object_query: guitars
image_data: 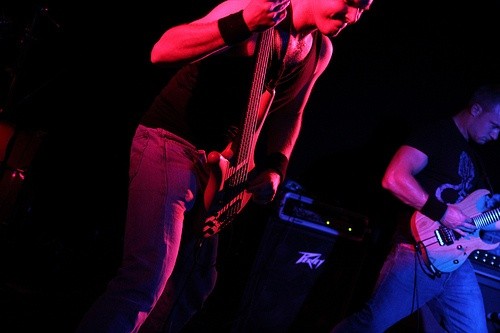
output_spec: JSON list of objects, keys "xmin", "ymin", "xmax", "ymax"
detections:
[
  {"xmin": 200, "ymin": 26, "xmax": 275, "ymax": 240},
  {"xmin": 410, "ymin": 189, "xmax": 500, "ymax": 274}
]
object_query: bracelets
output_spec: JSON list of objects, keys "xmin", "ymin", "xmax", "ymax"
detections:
[
  {"xmin": 217, "ymin": 10, "xmax": 253, "ymax": 47},
  {"xmin": 419, "ymin": 194, "xmax": 449, "ymax": 222},
  {"xmin": 262, "ymin": 151, "xmax": 289, "ymax": 182}
]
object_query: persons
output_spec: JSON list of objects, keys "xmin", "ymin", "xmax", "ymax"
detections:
[
  {"xmin": 330, "ymin": 79, "xmax": 500, "ymax": 333},
  {"xmin": 100, "ymin": 0, "xmax": 377, "ymax": 333}
]
